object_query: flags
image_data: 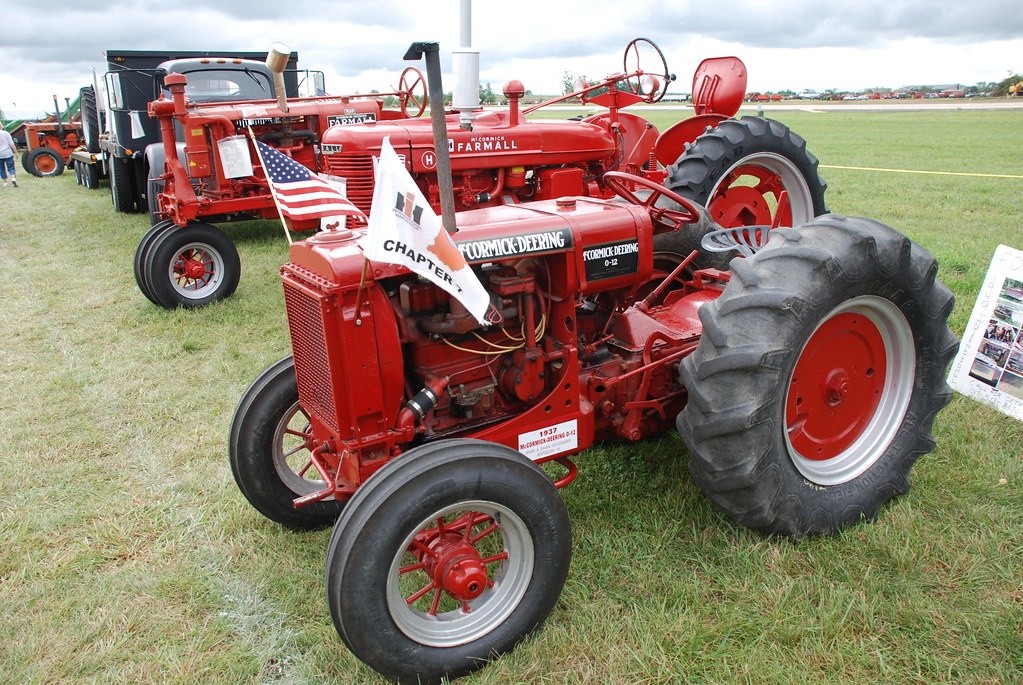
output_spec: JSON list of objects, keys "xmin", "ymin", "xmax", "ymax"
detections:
[
  {"xmin": 253, "ymin": 140, "xmax": 367, "ymax": 220},
  {"xmin": 363, "ymin": 136, "xmax": 492, "ymax": 327}
]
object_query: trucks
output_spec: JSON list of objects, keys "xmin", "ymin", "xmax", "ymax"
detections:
[{"xmin": 67, "ymin": 48, "xmax": 325, "ymax": 214}]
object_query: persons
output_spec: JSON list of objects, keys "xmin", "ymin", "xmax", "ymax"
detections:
[{"xmin": 0, "ymin": 120, "xmax": 20, "ymax": 189}]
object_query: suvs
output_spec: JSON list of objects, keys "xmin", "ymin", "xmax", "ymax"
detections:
[{"xmin": 132, "ymin": 51, "xmax": 429, "ymax": 311}]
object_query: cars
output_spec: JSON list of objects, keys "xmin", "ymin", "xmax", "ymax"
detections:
[{"xmin": 744, "ymin": 80, "xmax": 993, "ymax": 103}]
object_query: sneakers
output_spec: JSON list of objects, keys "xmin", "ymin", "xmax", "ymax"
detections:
[
  {"xmin": 3, "ymin": 183, "xmax": 9, "ymax": 187},
  {"xmin": 12, "ymin": 178, "xmax": 18, "ymax": 187}
]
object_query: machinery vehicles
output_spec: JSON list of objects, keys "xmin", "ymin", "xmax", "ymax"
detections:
[{"xmin": 226, "ymin": 36, "xmax": 962, "ymax": 684}]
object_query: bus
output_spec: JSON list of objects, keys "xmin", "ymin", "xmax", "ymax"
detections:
[{"xmin": 20, "ymin": 94, "xmax": 88, "ymax": 179}]
